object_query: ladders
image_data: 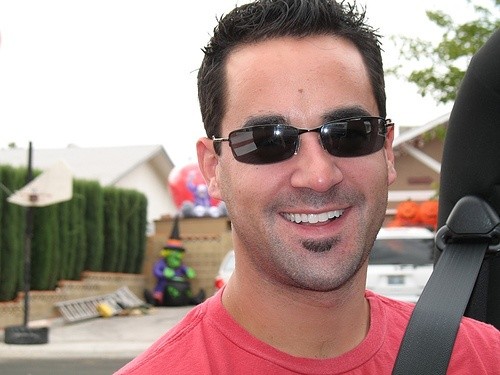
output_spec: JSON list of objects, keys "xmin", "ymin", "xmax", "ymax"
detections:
[{"xmin": 55, "ymin": 288, "xmax": 148, "ymax": 323}]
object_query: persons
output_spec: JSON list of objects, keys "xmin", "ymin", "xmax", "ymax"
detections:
[{"xmin": 110, "ymin": 0, "xmax": 500, "ymax": 375}]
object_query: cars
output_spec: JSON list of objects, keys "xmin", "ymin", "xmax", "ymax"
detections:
[{"xmin": 212, "ymin": 224, "xmax": 444, "ymax": 305}]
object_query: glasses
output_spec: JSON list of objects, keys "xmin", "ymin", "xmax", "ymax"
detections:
[{"xmin": 212, "ymin": 116, "xmax": 392, "ymax": 164}]
